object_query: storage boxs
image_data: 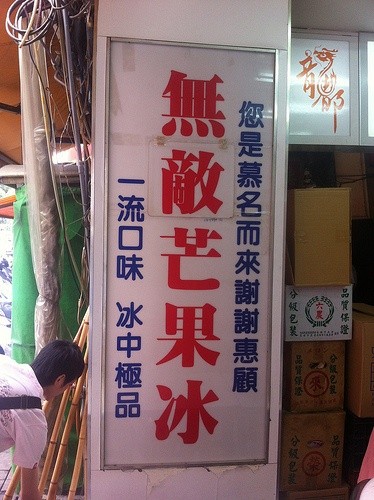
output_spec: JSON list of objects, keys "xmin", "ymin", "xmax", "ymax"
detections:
[{"xmin": 277, "ymin": 152, "xmax": 374, "ymax": 500}]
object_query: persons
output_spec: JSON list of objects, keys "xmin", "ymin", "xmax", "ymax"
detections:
[{"xmin": 0, "ymin": 339, "xmax": 85, "ymax": 500}]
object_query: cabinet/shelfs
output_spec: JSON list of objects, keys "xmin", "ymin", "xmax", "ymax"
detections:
[{"xmin": 288, "ymin": 25, "xmax": 374, "ymax": 147}]
